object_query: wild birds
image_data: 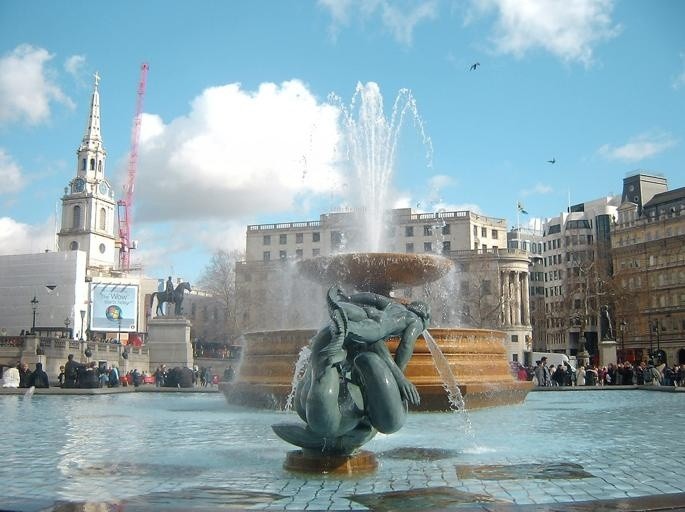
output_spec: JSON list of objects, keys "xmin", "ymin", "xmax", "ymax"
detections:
[
  {"xmin": 548, "ymin": 156, "xmax": 556, "ymax": 164},
  {"xmin": 469, "ymin": 62, "xmax": 480, "ymax": 72}
]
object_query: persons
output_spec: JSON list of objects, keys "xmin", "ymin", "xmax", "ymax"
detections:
[
  {"xmin": 0, "ymin": 327, "xmax": 241, "ymax": 388},
  {"xmin": 337, "ymin": 287, "xmax": 433, "ymax": 405},
  {"xmin": 166, "ymin": 276, "xmax": 175, "ymax": 304},
  {"xmin": 510, "ymin": 356, "xmax": 685, "ymax": 388},
  {"xmin": 601, "ymin": 304, "xmax": 616, "ymax": 341}
]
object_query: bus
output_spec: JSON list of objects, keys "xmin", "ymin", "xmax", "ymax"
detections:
[{"xmin": 591, "ymin": 346, "xmax": 642, "ymax": 365}]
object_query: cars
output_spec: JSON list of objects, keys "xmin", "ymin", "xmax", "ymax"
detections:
[{"xmin": 509, "ymin": 361, "xmax": 528, "ymax": 371}]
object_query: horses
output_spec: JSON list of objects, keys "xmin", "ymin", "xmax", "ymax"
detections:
[{"xmin": 150, "ymin": 281, "xmax": 192, "ymax": 316}]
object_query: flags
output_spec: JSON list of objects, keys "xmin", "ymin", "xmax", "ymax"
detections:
[{"xmin": 518, "ymin": 203, "xmax": 529, "ymax": 214}]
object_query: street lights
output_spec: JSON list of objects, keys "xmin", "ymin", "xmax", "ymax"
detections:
[
  {"xmin": 651, "ymin": 326, "xmax": 663, "ymax": 365},
  {"xmin": 618, "ymin": 317, "xmax": 626, "ymax": 363},
  {"xmin": 62, "ymin": 314, "xmax": 71, "ymax": 338},
  {"xmin": 78, "ymin": 307, "xmax": 87, "ymax": 340},
  {"xmin": 191, "ymin": 335, "xmax": 198, "ymax": 366},
  {"xmin": 115, "ymin": 310, "xmax": 123, "ymax": 342},
  {"xmin": 29, "ymin": 294, "xmax": 40, "ymax": 337},
  {"xmin": 647, "ymin": 318, "xmax": 655, "ymax": 364}
]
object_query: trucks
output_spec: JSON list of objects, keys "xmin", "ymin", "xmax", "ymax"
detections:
[{"xmin": 528, "ymin": 350, "xmax": 576, "ymax": 371}]
